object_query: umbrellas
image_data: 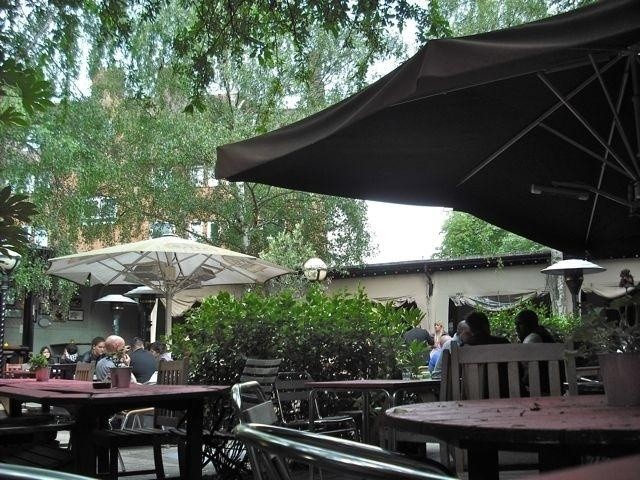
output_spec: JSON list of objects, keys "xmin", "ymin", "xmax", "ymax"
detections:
[
  {"xmin": 42, "ymin": 229, "xmax": 299, "ymax": 344},
  {"xmin": 214, "ymin": 0, "xmax": 640, "ymax": 262}
]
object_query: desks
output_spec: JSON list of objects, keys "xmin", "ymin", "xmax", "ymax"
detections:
[{"xmin": 383, "ymin": 395, "xmax": 640, "ymax": 480}]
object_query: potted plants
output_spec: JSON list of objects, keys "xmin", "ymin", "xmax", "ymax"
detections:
[{"xmin": 567, "ymin": 306, "xmax": 640, "ymax": 405}]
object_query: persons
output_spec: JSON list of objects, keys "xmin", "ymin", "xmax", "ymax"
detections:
[
  {"xmin": 38, "ymin": 335, "xmax": 176, "ymax": 389},
  {"xmin": 431, "ymin": 319, "xmax": 472, "ymax": 378},
  {"xmin": 460, "ymin": 313, "xmax": 524, "ymax": 400},
  {"xmin": 403, "ymin": 324, "xmax": 434, "ymax": 351},
  {"xmin": 429, "ymin": 336, "xmax": 450, "ymax": 373},
  {"xmin": 514, "ymin": 309, "xmax": 566, "ymax": 397},
  {"xmin": 433, "ymin": 321, "xmax": 451, "ymax": 352}
]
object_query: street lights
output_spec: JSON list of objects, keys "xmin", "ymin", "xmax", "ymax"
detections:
[
  {"xmin": 0, "ymin": 245, "xmax": 22, "ymax": 355},
  {"xmin": 541, "ymin": 257, "xmax": 608, "ymax": 324},
  {"xmin": 21, "ymin": 225, "xmax": 48, "ymax": 349}
]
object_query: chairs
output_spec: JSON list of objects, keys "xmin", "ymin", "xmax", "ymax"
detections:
[
  {"xmin": 380, "ymin": 349, "xmax": 450, "ymax": 470},
  {"xmin": 451, "ymin": 340, "xmax": 577, "ymax": 480}
]
